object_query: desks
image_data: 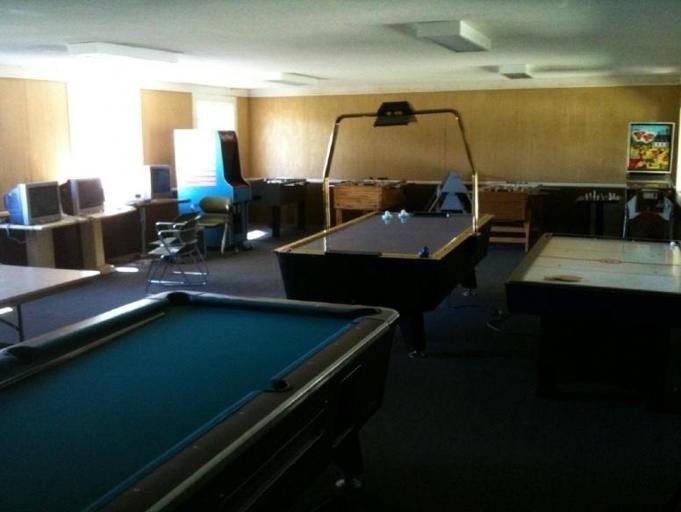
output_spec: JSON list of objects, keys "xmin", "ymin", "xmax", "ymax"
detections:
[
  {"xmin": 128, "ymin": 198, "xmax": 189, "ymax": 243},
  {"xmin": 80, "ymin": 206, "xmax": 136, "ymax": 274},
  {"xmin": 0, "ymin": 215, "xmax": 87, "ymax": 269},
  {"xmin": 0, "ymin": 262, "xmax": 101, "ymax": 349}
]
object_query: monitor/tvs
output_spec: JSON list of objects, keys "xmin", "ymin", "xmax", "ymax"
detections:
[
  {"xmin": 2, "ymin": 180, "xmax": 63, "ymax": 226},
  {"xmin": 141, "ymin": 165, "xmax": 172, "ymax": 200},
  {"xmin": 59, "ymin": 177, "xmax": 105, "ymax": 216}
]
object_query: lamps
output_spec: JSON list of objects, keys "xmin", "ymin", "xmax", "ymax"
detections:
[{"xmin": 68, "ymin": 42, "xmax": 180, "ymax": 69}]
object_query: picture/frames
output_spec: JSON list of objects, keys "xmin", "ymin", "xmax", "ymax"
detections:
[{"xmin": 626, "ymin": 122, "xmax": 676, "ymax": 174}]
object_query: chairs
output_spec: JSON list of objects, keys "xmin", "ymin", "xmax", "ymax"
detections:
[
  {"xmin": 190, "ymin": 196, "xmax": 239, "ymax": 255},
  {"xmin": 147, "ymin": 213, "xmax": 210, "ymax": 274},
  {"xmin": 145, "ymin": 215, "xmax": 210, "ymax": 290}
]
